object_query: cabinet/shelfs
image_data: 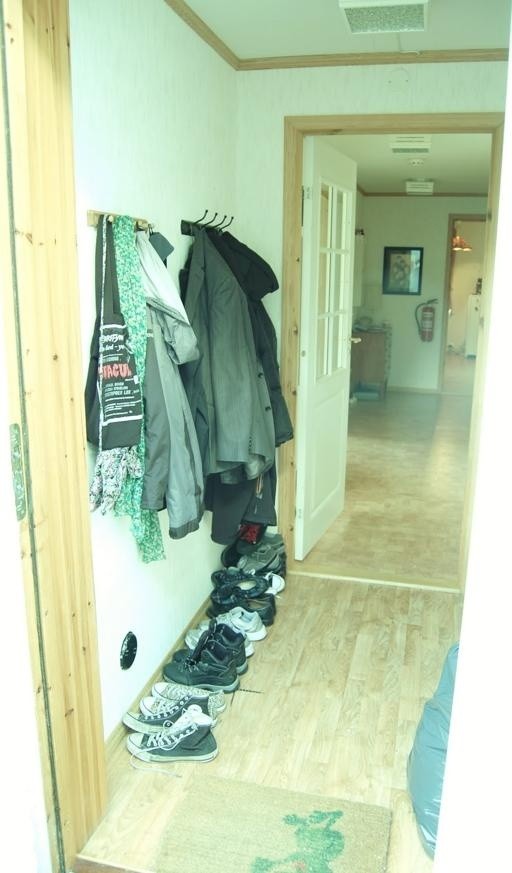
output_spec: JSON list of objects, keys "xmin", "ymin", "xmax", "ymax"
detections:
[{"xmin": 350, "ymin": 331, "xmax": 391, "ymax": 399}]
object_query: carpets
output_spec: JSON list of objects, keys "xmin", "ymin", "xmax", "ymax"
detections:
[{"xmin": 150, "ymin": 774, "xmax": 392, "ymax": 873}]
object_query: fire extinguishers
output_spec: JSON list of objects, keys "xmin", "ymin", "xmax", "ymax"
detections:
[{"xmin": 415, "ymin": 299, "xmax": 438, "ymax": 342}]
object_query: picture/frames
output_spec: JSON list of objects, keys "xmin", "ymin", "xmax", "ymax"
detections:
[{"xmin": 382, "ymin": 247, "xmax": 423, "ymax": 295}]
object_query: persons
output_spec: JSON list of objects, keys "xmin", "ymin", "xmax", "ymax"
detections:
[{"xmin": 392, "ymin": 255, "xmax": 410, "ymax": 289}]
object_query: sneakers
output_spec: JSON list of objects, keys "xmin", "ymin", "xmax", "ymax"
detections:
[{"xmin": 122, "ymin": 523, "xmax": 287, "ymax": 763}]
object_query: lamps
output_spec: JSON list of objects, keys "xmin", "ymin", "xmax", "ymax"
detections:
[{"xmin": 452, "ymin": 235, "xmax": 472, "ymax": 252}]
